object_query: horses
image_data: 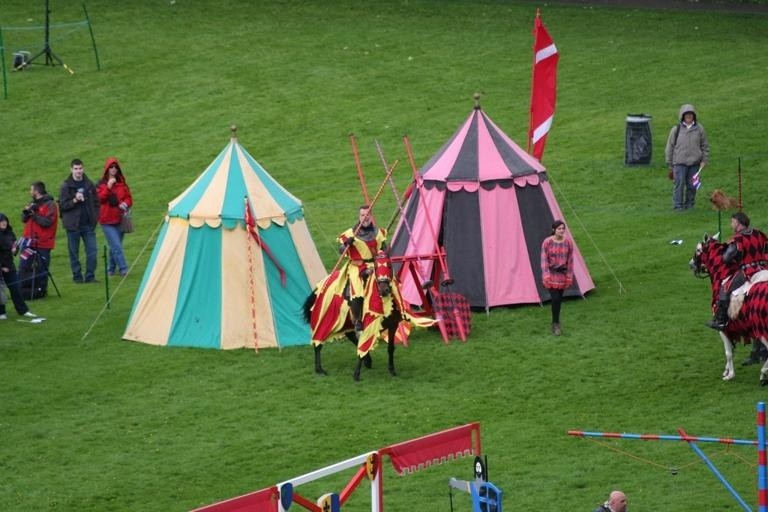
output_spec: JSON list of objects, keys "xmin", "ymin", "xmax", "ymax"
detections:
[
  {"xmin": 689, "ymin": 231, "xmax": 768, "ymax": 386},
  {"xmin": 293, "ymin": 243, "xmax": 402, "ymax": 381}
]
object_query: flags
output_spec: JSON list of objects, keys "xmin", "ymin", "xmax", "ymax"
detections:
[
  {"xmin": 530, "ymin": 18, "xmax": 559, "ymax": 162},
  {"xmin": 693, "ymin": 174, "xmax": 702, "ymax": 190},
  {"xmin": 20, "ymin": 248, "xmax": 34, "ymax": 261},
  {"xmin": 18, "ymin": 237, "xmax": 32, "ymax": 247}
]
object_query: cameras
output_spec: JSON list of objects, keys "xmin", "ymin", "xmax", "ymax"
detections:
[{"xmin": 22, "ymin": 203, "xmax": 36, "ymax": 214}]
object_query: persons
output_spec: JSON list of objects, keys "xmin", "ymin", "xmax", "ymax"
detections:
[
  {"xmin": 705, "ymin": 213, "xmax": 768, "ymax": 331},
  {"xmin": 595, "ymin": 490, "xmax": 627, "ymax": 512},
  {"xmin": 94, "ymin": 157, "xmax": 133, "ymax": 277},
  {"xmin": 339, "ymin": 205, "xmax": 389, "ymax": 330},
  {"xmin": 58, "ymin": 159, "xmax": 100, "ymax": 285},
  {"xmin": 664, "ymin": 105, "xmax": 710, "ymax": 212},
  {"xmin": 17, "ymin": 181, "xmax": 58, "ymax": 297},
  {"xmin": 0, "ymin": 214, "xmax": 36, "ymax": 320},
  {"xmin": 540, "ymin": 220, "xmax": 574, "ymax": 336}
]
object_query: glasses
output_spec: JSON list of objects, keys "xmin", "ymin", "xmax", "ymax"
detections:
[{"xmin": 109, "ymin": 164, "xmax": 119, "ymax": 169}]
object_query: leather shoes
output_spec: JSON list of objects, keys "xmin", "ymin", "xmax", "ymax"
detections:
[{"xmin": 741, "ymin": 360, "xmax": 759, "ymax": 366}]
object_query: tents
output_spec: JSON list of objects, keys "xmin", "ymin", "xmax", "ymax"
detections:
[
  {"xmin": 387, "ymin": 94, "xmax": 597, "ymax": 308},
  {"xmin": 120, "ymin": 125, "xmax": 330, "ymax": 351}
]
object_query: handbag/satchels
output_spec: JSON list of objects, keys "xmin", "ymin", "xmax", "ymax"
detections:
[{"xmin": 119, "ymin": 208, "xmax": 134, "ymax": 234}]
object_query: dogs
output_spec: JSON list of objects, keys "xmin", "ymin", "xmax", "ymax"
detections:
[{"xmin": 709, "ymin": 189, "xmax": 743, "ymax": 210}]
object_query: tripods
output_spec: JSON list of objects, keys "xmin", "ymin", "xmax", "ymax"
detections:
[
  {"xmin": 30, "ymin": 214, "xmax": 61, "ymax": 300},
  {"xmin": 11, "ymin": 0, "xmax": 75, "ymax": 74}
]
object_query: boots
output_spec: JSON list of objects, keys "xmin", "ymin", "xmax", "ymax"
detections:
[{"xmin": 711, "ymin": 300, "xmax": 727, "ymax": 327}]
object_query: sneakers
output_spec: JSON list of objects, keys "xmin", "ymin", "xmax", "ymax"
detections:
[
  {"xmin": 0, "ymin": 314, "xmax": 6, "ymax": 320},
  {"xmin": 553, "ymin": 323, "xmax": 560, "ymax": 336},
  {"xmin": 23, "ymin": 312, "xmax": 36, "ymax": 317},
  {"xmin": 73, "ymin": 275, "xmax": 82, "ymax": 281},
  {"xmin": 120, "ymin": 271, "xmax": 126, "ymax": 276},
  {"xmin": 108, "ymin": 271, "xmax": 113, "ymax": 276},
  {"xmin": 85, "ymin": 275, "xmax": 93, "ymax": 282}
]
